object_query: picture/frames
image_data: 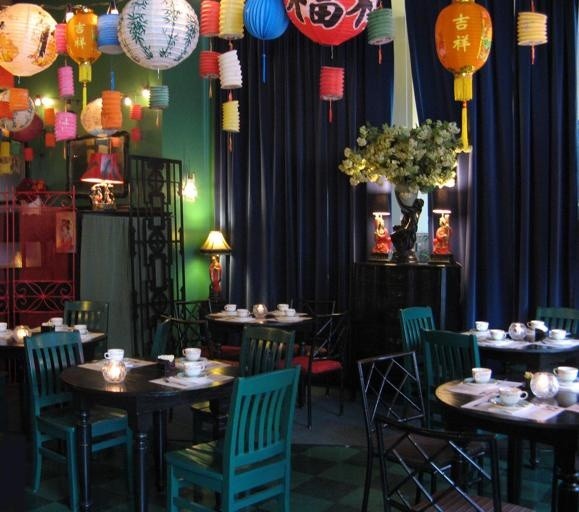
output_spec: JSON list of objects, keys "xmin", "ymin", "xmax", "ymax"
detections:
[{"xmin": 65, "ymin": 131, "xmax": 129, "ymax": 200}]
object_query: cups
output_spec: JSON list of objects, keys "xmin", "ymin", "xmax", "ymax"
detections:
[
  {"xmin": 552, "ymin": 366, "xmax": 579, "ymax": 383},
  {"xmin": 224, "ymin": 304, "xmax": 296, "ymax": 317},
  {"xmin": 0, "ymin": 317, "xmax": 87, "ymax": 337},
  {"xmin": 183, "ymin": 347, "xmax": 201, "ymax": 361},
  {"xmin": 472, "ymin": 368, "xmax": 491, "ymax": 383},
  {"xmin": 475, "ymin": 320, "xmax": 567, "ymax": 340},
  {"xmin": 104, "ymin": 348, "xmax": 125, "ymax": 362},
  {"xmin": 497, "ymin": 385, "xmax": 528, "ymax": 405},
  {"xmin": 184, "ymin": 361, "xmax": 205, "ymax": 376}
]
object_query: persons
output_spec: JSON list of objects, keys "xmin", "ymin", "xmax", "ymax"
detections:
[
  {"xmin": 208, "ymin": 256, "xmax": 223, "ymax": 298},
  {"xmin": 434, "ymin": 215, "xmax": 452, "ymax": 253},
  {"xmin": 374, "ymin": 216, "xmax": 391, "ymax": 254}
]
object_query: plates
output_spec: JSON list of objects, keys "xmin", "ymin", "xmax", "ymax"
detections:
[
  {"xmin": 177, "ymin": 372, "xmax": 206, "ymax": 380},
  {"xmin": 489, "ymin": 395, "xmax": 531, "ymax": 410},
  {"xmin": 464, "ymin": 377, "xmax": 497, "ymax": 388}
]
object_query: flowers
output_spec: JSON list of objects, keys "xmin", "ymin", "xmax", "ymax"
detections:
[{"xmin": 339, "ymin": 118, "xmax": 464, "ymax": 194}]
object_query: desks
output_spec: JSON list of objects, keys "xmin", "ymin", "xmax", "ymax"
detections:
[{"xmin": 347, "ymin": 261, "xmax": 465, "ymax": 404}]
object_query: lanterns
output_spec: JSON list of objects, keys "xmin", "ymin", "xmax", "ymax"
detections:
[{"xmin": 0, "ymin": 1, "xmax": 557, "ymax": 154}]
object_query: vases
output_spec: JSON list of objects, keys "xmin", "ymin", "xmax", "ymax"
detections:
[{"xmin": 394, "ymin": 176, "xmax": 417, "ymax": 208}]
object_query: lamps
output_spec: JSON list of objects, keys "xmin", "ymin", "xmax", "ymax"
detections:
[
  {"xmin": 34, "ymin": 94, "xmax": 41, "ymax": 105},
  {"xmin": 124, "ymin": 96, "xmax": 132, "ymax": 106},
  {"xmin": 143, "ymin": 87, "xmax": 151, "ymax": 98},
  {"xmin": 199, "ymin": 230, "xmax": 232, "ymax": 302},
  {"xmin": 64, "ymin": 99, "xmax": 72, "ymax": 112},
  {"xmin": 80, "ymin": 152, "xmax": 127, "ymax": 211},
  {"xmin": 182, "ymin": 173, "xmax": 199, "ymax": 203},
  {"xmin": 111, "ymin": 1, "xmax": 119, "ymax": 14},
  {"xmin": 427, "ymin": 188, "xmax": 454, "ymax": 264},
  {"xmin": 65, "ymin": 3, "xmax": 74, "ymax": 24},
  {"xmin": 368, "ymin": 194, "xmax": 392, "ymax": 262}
]
